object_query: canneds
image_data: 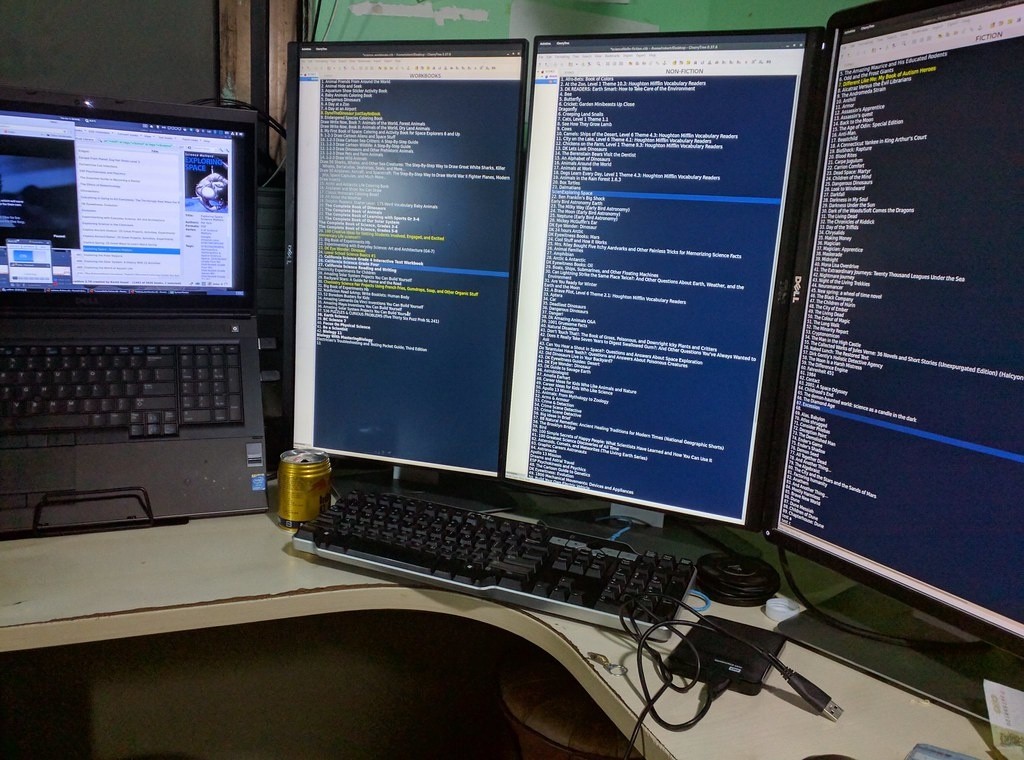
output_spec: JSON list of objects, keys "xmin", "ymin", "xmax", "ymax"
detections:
[{"xmin": 276, "ymin": 449, "xmax": 332, "ymax": 533}]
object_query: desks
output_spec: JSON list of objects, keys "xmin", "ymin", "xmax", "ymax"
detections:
[{"xmin": 0, "ymin": 466, "xmax": 1005, "ymax": 760}]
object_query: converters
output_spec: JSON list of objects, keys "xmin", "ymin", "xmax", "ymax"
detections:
[{"xmin": 668, "ymin": 615, "xmax": 788, "ymax": 697}]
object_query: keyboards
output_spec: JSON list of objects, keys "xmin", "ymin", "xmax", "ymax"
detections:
[{"xmin": 291, "ymin": 488, "xmax": 698, "ymax": 642}]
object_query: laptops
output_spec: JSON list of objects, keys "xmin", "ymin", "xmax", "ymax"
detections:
[{"xmin": 0, "ymin": 83, "xmax": 271, "ymax": 541}]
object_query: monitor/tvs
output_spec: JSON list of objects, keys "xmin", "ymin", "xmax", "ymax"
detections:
[
  {"xmin": 499, "ymin": 25, "xmax": 827, "ymax": 534},
  {"xmin": 282, "ymin": 38, "xmax": 530, "ymax": 481},
  {"xmin": 759, "ymin": 0, "xmax": 1024, "ymax": 662}
]
